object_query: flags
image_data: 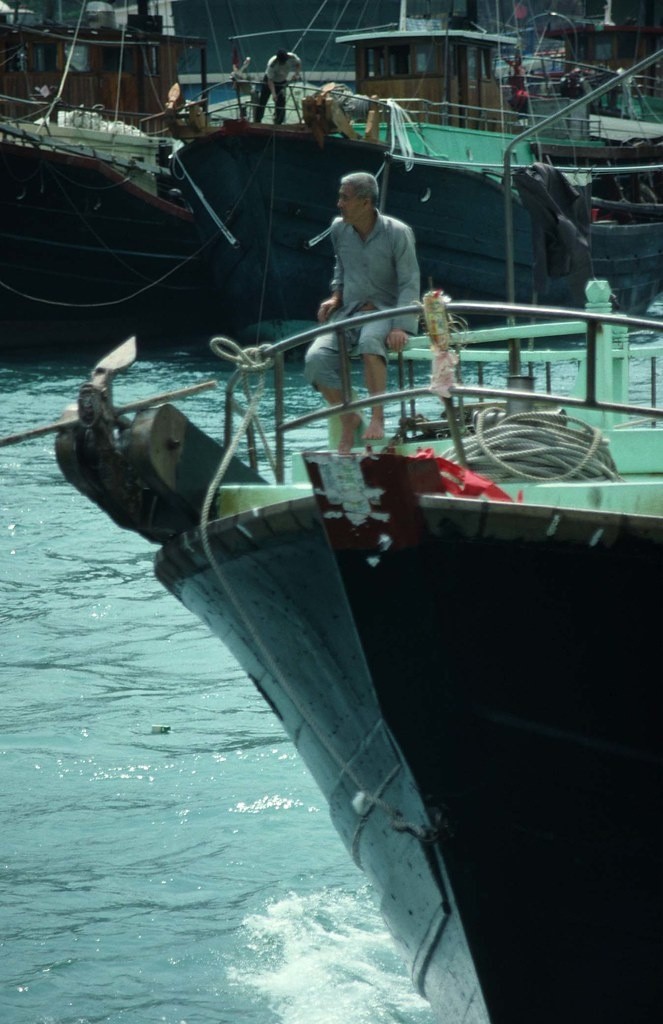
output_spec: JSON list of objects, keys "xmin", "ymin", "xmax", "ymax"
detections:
[{"xmin": 514, "ymin": 3, "xmax": 526, "ymax": 18}]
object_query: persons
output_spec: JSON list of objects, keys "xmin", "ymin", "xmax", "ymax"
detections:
[
  {"xmin": 254, "ymin": 48, "xmax": 301, "ymax": 125},
  {"xmin": 503, "ymin": 56, "xmax": 523, "ymax": 74},
  {"xmin": 305, "ymin": 172, "xmax": 420, "ymax": 454}
]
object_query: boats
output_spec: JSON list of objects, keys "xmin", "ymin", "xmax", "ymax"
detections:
[
  {"xmin": 55, "ymin": 301, "xmax": 663, "ymax": 1024},
  {"xmin": 0, "ymin": 0, "xmax": 355, "ymax": 345},
  {"xmin": 169, "ymin": 0, "xmax": 663, "ymax": 357}
]
